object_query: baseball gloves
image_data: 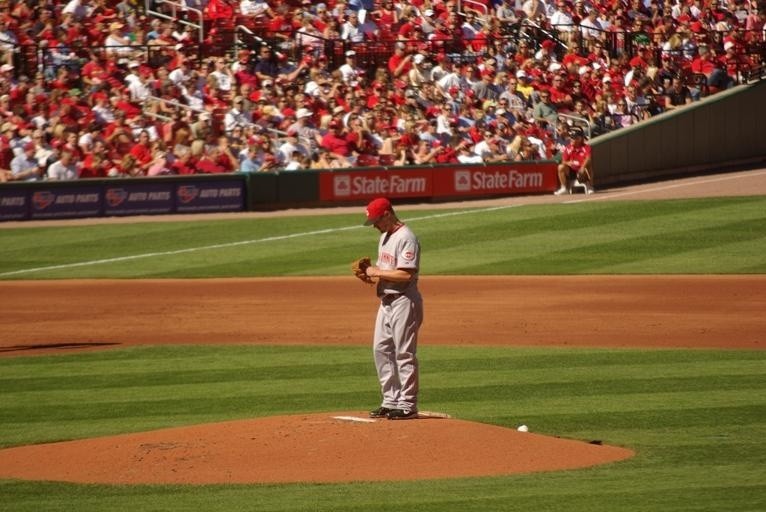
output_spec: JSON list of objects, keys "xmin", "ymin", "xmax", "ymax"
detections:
[
  {"xmin": 351, "ymin": 256, "xmax": 376, "ymax": 284},
  {"xmin": 577, "ymin": 168, "xmax": 590, "ymax": 185}
]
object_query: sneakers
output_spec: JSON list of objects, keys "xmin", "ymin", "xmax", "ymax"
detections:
[
  {"xmin": 369, "ymin": 406, "xmax": 396, "ymax": 418},
  {"xmin": 588, "ymin": 188, "xmax": 595, "ymax": 194},
  {"xmin": 386, "ymin": 410, "xmax": 419, "ymax": 420},
  {"xmin": 554, "ymin": 189, "xmax": 568, "ymax": 196}
]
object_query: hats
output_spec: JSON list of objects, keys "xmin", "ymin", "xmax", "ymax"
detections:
[
  {"xmin": 362, "ymin": 197, "xmax": 392, "ymax": 226},
  {"xmin": 1, "ymin": 0, "xmax": 736, "ymax": 157}
]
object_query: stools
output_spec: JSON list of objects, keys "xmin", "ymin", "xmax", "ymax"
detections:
[{"xmin": 568, "ymin": 174, "xmax": 593, "ymax": 195}]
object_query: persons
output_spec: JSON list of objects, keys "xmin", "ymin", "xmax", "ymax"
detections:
[
  {"xmin": 0, "ymin": 1, "xmax": 765, "ymax": 182},
  {"xmin": 354, "ymin": 198, "xmax": 424, "ymax": 420},
  {"xmin": 552, "ymin": 125, "xmax": 594, "ymax": 195}
]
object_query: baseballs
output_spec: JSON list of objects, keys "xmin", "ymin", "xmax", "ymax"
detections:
[{"xmin": 517, "ymin": 425, "xmax": 528, "ymax": 432}]
